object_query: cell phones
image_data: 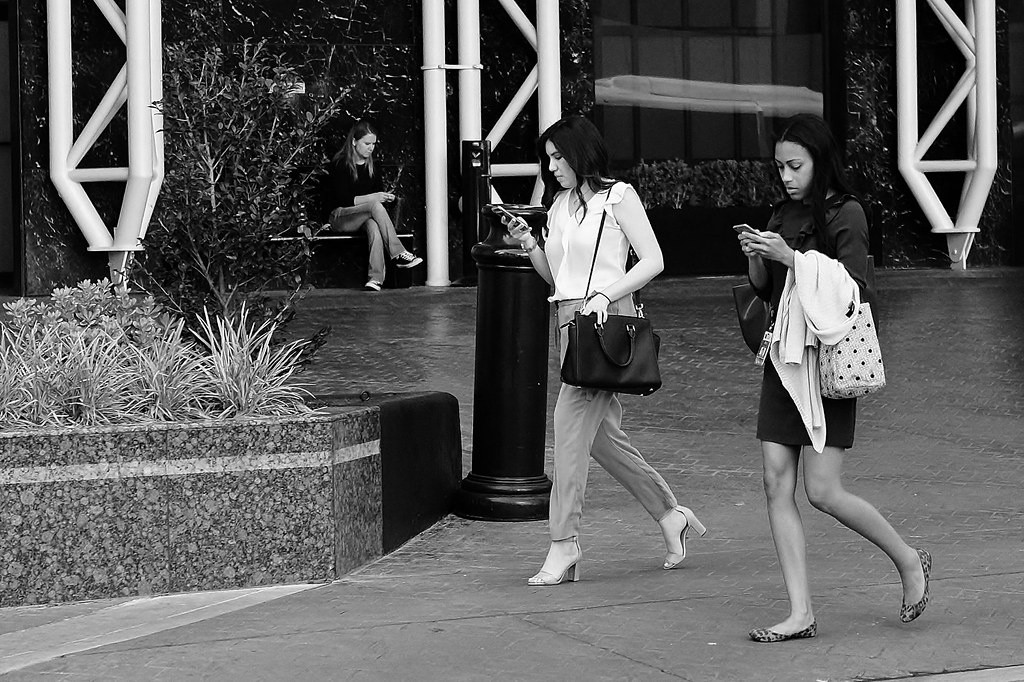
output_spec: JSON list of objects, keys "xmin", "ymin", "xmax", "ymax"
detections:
[
  {"xmin": 733, "ymin": 224, "xmax": 761, "ymax": 237},
  {"xmin": 491, "ymin": 206, "xmax": 529, "ymax": 231}
]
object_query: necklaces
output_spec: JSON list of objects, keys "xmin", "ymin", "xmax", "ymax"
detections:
[{"xmin": 571, "ymin": 189, "xmax": 592, "ymax": 204}]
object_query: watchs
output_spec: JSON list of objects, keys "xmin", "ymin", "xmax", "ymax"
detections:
[{"xmin": 520, "ymin": 238, "xmax": 537, "ymax": 252}]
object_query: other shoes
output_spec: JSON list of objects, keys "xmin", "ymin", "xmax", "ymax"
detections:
[
  {"xmin": 748, "ymin": 618, "xmax": 817, "ymax": 642},
  {"xmin": 899, "ymin": 548, "xmax": 931, "ymax": 623}
]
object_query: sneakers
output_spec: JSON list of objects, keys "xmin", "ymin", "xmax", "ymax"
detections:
[
  {"xmin": 365, "ymin": 279, "xmax": 382, "ymax": 291},
  {"xmin": 396, "ymin": 250, "xmax": 424, "ymax": 269}
]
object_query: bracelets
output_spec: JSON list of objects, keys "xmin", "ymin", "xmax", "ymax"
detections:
[{"xmin": 598, "ymin": 292, "xmax": 612, "ymax": 304}]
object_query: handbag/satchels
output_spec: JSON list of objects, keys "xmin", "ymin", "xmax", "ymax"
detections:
[
  {"xmin": 820, "ymin": 299, "xmax": 887, "ymax": 400},
  {"xmin": 732, "ymin": 283, "xmax": 770, "ymax": 355},
  {"xmin": 560, "ymin": 309, "xmax": 663, "ymax": 396}
]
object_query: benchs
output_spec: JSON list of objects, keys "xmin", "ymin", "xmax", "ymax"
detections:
[{"xmin": 191, "ymin": 232, "xmax": 416, "ymax": 289}]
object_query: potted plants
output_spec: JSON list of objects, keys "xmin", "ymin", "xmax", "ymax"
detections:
[{"xmin": 629, "ymin": 158, "xmax": 884, "ymax": 279}]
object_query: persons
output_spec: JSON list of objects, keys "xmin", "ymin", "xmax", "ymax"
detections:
[
  {"xmin": 500, "ymin": 118, "xmax": 707, "ymax": 586},
  {"xmin": 737, "ymin": 112, "xmax": 932, "ymax": 643},
  {"xmin": 322, "ymin": 122, "xmax": 424, "ymax": 292}
]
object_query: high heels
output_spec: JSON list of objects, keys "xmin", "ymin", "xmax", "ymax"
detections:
[
  {"xmin": 662, "ymin": 505, "xmax": 708, "ymax": 570},
  {"xmin": 527, "ymin": 537, "xmax": 583, "ymax": 585}
]
